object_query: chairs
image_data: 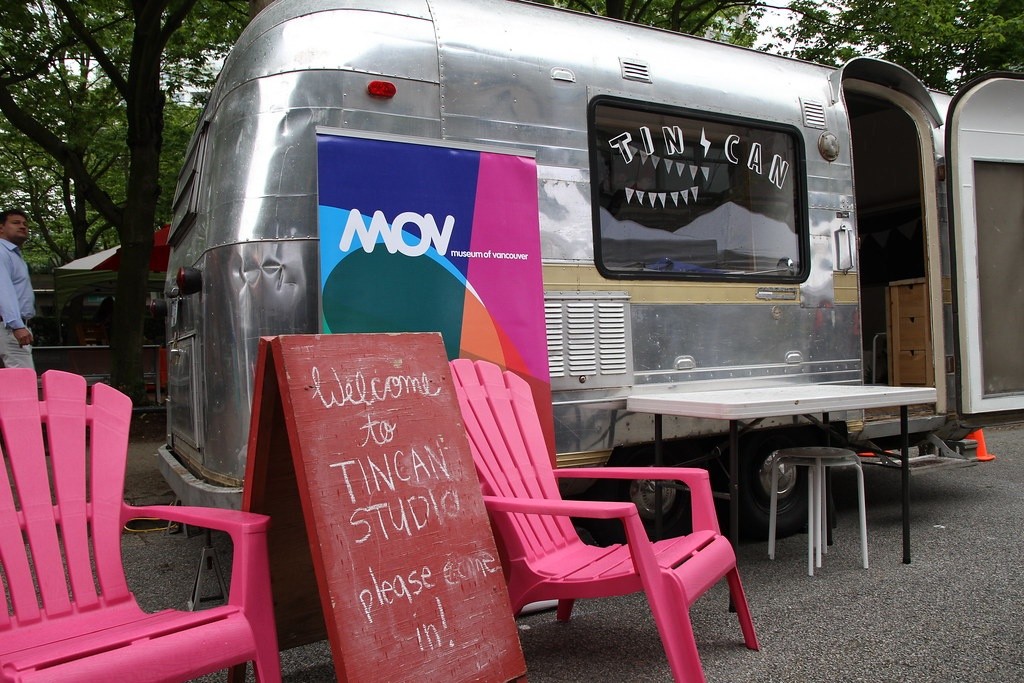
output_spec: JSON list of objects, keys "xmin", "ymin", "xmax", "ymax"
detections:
[
  {"xmin": 0, "ymin": 368, "xmax": 283, "ymax": 683},
  {"xmin": 444, "ymin": 359, "xmax": 759, "ymax": 683}
]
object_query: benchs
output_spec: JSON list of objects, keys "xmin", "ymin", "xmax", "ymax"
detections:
[{"xmin": 27, "ymin": 345, "xmax": 162, "ymax": 405}]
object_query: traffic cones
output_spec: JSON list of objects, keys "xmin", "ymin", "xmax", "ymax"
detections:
[{"xmin": 964, "ymin": 427, "xmax": 996, "ymax": 462}]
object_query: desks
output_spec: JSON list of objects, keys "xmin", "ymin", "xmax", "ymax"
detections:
[{"xmin": 627, "ymin": 384, "xmax": 937, "ymax": 612}]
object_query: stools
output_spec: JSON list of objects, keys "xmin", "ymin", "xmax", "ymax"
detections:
[{"xmin": 768, "ymin": 447, "xmax": 868, "ymax": 576}]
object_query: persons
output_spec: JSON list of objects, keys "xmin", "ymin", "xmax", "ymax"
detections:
[
  {"xmin": 0, "ymin": 208, "xmax": 36, "ymax": 370},
  {"xmin": 94, "ymin": 297, "xmax": 114, "ymax": 345}
]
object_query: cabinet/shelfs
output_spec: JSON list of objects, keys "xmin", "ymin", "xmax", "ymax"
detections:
[{"xmin": 885, "ymin": 277, "xmax": 934, "ymax": 387}]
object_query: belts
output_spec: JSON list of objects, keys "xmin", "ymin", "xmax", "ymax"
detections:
[{"xmin": 0, "ymin": 315, "xmax": 30, "ymax": 327}]
